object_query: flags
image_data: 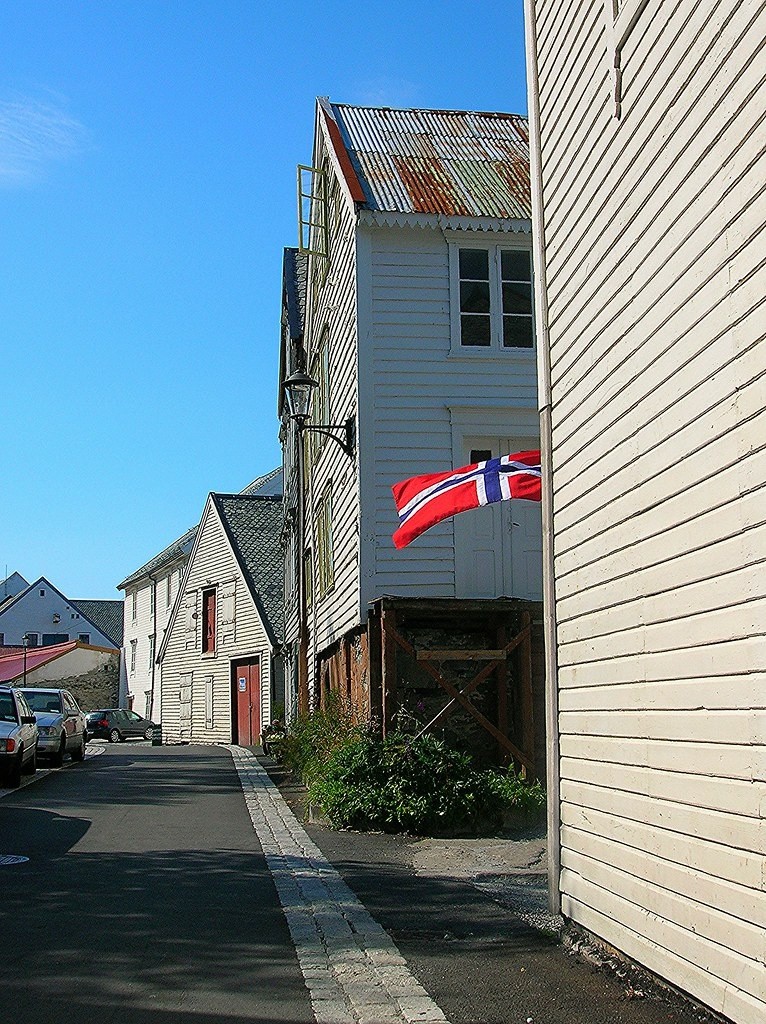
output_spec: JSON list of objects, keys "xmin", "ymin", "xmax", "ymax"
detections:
[{"xmin": 392, "ymin": 448, "xmax": 542, "ymax": 550}]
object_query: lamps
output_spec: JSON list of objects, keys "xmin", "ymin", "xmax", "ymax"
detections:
[{"xmin": 278, "ymin": 366, "xmax": 355, "ymax": 456}]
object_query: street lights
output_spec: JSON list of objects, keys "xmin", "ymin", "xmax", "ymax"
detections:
[
  {"xmin": 282, "ymin": 358, "xmax": 320, "ymax": 722},
  {"xmin": 22, "ymin": 633, "xmax": 30, "ymax": 687}
]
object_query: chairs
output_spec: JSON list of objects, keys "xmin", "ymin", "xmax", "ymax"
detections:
[
  {"xmin": 0, "ymin": 700, "xmax": 13, "ymax": 720},
  {"xmin": 46, "ymin": 701, "xmax": 62, "ymax": 714}
]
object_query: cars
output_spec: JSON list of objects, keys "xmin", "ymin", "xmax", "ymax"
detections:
[
  {"xmin": 0, "ymin": 686, "xmax": 38, "ymax": 787},
  {"xmin": 86, "ymin": 709, "xmax": 155, "ymax": 743},
  {"xmin": 19, "ymin": 688, "xmax": 85, "ymax": 767}
]
